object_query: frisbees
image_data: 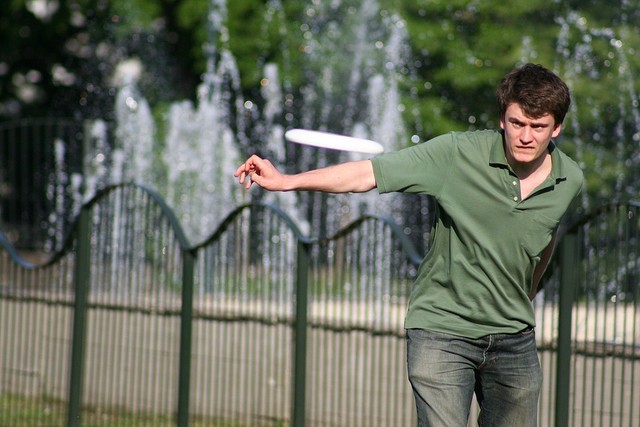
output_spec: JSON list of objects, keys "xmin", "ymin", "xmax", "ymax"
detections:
[{"xmin": 285, "ymin": 128, "xmax": 384, "ymax": 154}]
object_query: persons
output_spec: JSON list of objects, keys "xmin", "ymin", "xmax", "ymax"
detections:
[{"xmin": 234, "ymin": 64, "xmax": 585, "ymax": 427}]
